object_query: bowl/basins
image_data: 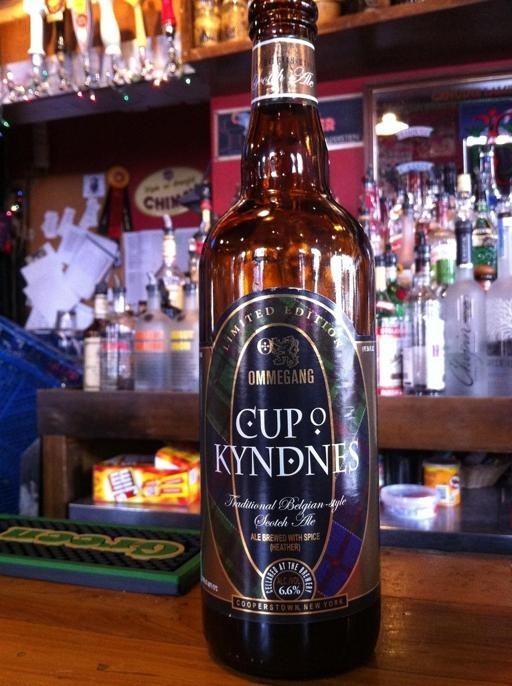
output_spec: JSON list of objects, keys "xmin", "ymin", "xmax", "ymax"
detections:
[{"xmin": 377, "ymin": 483, "xmax": 442, "ymax": 520}]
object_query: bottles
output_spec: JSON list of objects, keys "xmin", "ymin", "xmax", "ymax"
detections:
[
  {"xmin": 76, "ymin": 144, "xmax": 512, "ymax": 398},
  {"xmin": 195, "ymin": 1, "xmax": 382, "ymax": 685},
  {"xmin": 421, "ymin": 456, "xmax": 462, "ymax": 508}
]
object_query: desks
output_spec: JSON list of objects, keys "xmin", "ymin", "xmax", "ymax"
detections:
[{"xmin": 1, "ymin": 543, "xmax": 511, "ymax": 686}]
